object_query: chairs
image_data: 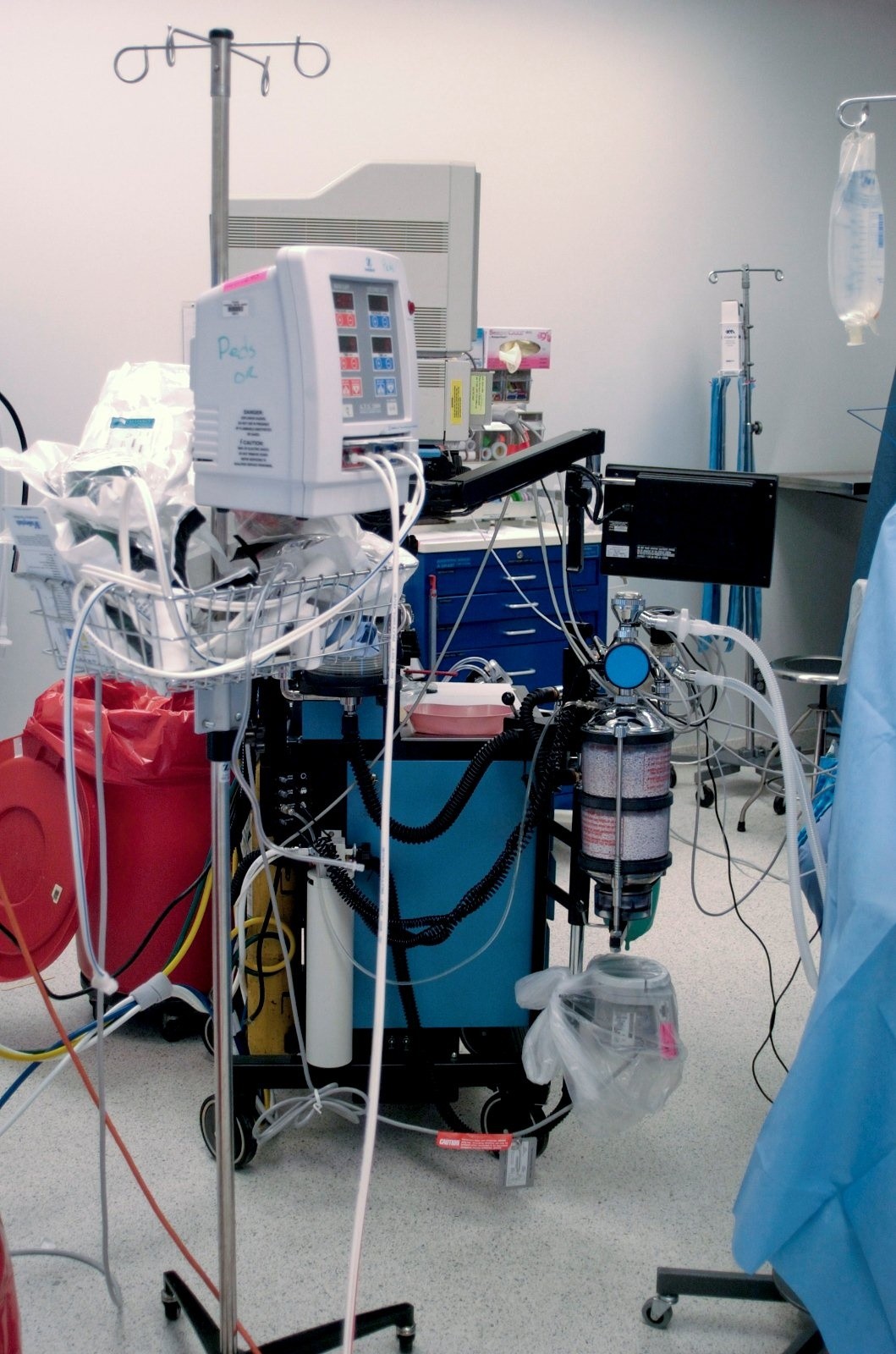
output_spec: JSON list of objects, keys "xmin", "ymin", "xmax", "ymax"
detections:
[{"xmin": 737, "ymin": 578, "xmax": 867, "ymax": 830}]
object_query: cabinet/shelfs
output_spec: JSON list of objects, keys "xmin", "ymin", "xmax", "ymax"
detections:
[{"xmin": 400, "ymin": 543, "xmax": 608, "ymax": 809}]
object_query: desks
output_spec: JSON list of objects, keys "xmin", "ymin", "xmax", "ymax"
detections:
[{"xmin": 777, "ymin": 471, "xmax": 872, "ymax": 495}]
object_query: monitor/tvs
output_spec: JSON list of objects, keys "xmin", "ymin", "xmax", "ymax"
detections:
[
  {"xmin": 189, "ymin": 247, "xmax": 416, "ymax": 430},
  {"xmin": 228, "ymin": 161, "xmax": 482, "ymax": 351}
]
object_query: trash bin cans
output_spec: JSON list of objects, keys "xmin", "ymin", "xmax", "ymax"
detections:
[{"xmin": 0, "ymin": 678, "xmax": 212, "ymax": 1044}]
